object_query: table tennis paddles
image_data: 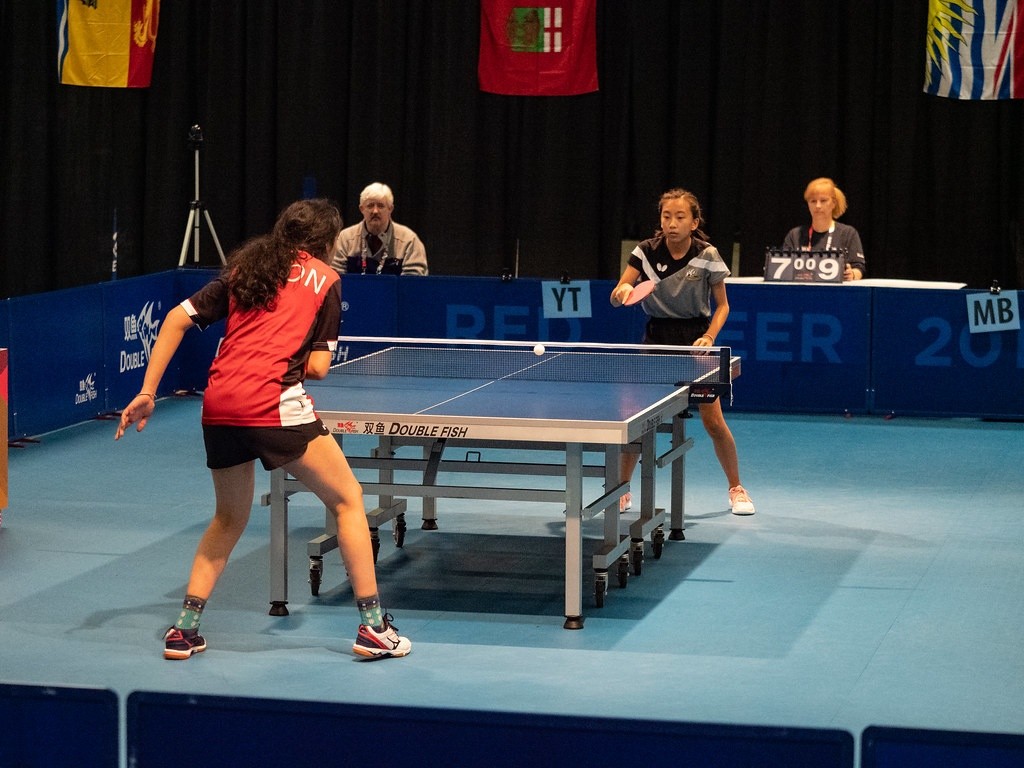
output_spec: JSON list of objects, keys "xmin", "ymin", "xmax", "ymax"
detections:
[{"xmin": 623, "ymin": 279, "xmax": 656, "ymax": 307}]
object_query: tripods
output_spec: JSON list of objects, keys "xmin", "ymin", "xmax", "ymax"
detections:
[{"xmin": 179, "ymin": 125, "xmax": 229, "ymax": 271}]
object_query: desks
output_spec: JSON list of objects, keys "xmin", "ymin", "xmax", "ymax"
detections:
[
  {"xmin": 723, "ymin": 277, "xmax": 967, "ymax": 289},
  {"xmin": 261, "ymin": 348, "xmax": 741, "ymax": 630}
]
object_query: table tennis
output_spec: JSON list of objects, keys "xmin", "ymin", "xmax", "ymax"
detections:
[{"xmin": 533, "ymin": 344, "xmax": 546, "ymax": 356}]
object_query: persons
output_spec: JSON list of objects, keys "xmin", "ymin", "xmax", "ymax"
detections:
[
  {"xmin": 114, "ymin": 197, "xmax": 412, "ymax": 660},
  {"xmin": 603, "ymin": 187, "xmax": 757, "ymax": 517},
  {"xmin": 783, "ymin": 177, "xmax": 866, "ymax": 281},
  {"xmin": 329, "ymin": 181, "xmax": 429, "ymax": 277}
]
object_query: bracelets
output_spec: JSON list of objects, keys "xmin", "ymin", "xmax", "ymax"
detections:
[
  {"xmin": 136, "ymin": 393, "xmax": 157, "ymax": 401},
  {"xmin": 703, "ymin": 333, "xmax": 714, "ymax": 345},
  {"xmin": 851, "ymin": 270, "xmax": 855, "ymax": 280}
]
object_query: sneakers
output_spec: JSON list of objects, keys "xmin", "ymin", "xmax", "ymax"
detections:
[
  {"xmin": 728, "ymin": 484, "xmax": 755, "ymax": 515},
  {"xmin": 162, "ymin": 625, "xmax": 206, "ymax": 659},
  {"xmin": 618, "ymin": 491, "xmax": 631, "ymax": 512},
  {"xmin": 353, "ymin": 613, "xmax": 411, "ymax": 658}
]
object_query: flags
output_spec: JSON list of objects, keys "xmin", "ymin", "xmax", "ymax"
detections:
[
  {"xmin": 478, "ymin": 0, "xmax": 599, "ymax": 96},
  {"xmin": 922, "ymin": 0, "xmax": 1024, "ymax": 100},
  {"xmin": 56, "ymin": 0, "xmax": 160, "ymax": 88}
]
